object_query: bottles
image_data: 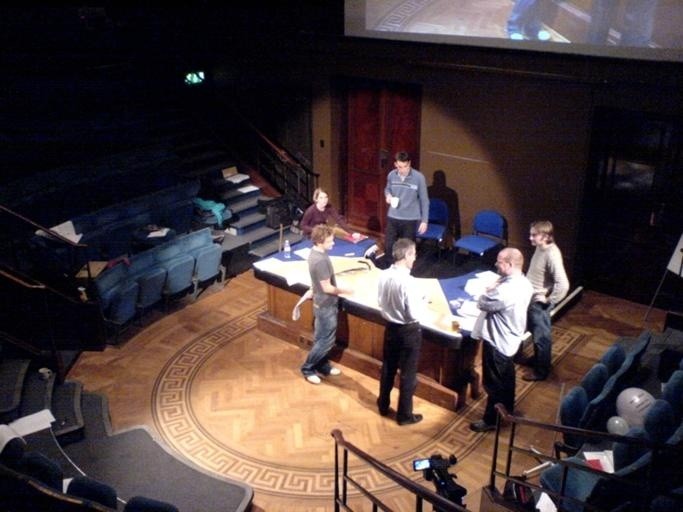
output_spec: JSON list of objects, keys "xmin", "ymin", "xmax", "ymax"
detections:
[
  {"xmin": 451, "ymin": 316, "xmax": 459, "ymax": 332},
  {"xmin": 77, "ymin": 287, "xmax": 88, "ymax": 303},
  {"xmin": 283, "ymin": 240, "xmax": 290, "ymax": 259}
]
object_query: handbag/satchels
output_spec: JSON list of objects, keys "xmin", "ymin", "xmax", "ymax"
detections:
[{"xmin": 265, "ymin": 199, "xmax": 292, "ymax": 229}]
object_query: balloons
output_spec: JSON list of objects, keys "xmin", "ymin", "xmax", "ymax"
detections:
[
  {"xmin": 617, "ymin": 387, "xmax": 657, "ymax": 428},
  {"xmin": 606, "ymin": 417, "xmax": 631, "ymax": 435}
]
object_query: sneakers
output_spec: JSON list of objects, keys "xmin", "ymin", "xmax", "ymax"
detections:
[
  {"xmin": 329, "ymin": 367, "xmax": 340, "ymax": 375},
  {"xmin": 306, "ymin": 374, "xmax": 321, "ymax": 384}
]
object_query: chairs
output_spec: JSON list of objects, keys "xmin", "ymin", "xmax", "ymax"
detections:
[
  {"xmin": 451, "ymin": 210, "xmax": 512, "ymax": 262},
  {"xmin": 1, "ymin": 150, "xmax": 228, "ymax": 346},
  {"xmin": 536, "ymin": 339, "xmax": 683, "ymax": 512},
  {"xmin": 2, "ymin": 437, "xmax": 178, "ymax": 512},
  {"xmin": 415, "ymin": 199, "xmax": 451, "ymax": 258}
]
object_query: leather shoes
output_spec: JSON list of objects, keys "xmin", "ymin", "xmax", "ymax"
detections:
[
  {"xmin": 470, "ymin": 419, "xmax": 496, "ymax": 432},
  {"xmin": 397, "ymin": 414, "xmax": 422, "ymax": 425},
  {"xmin": 522, "ymin": 371, "xmax": 546, "ymax": 380}
]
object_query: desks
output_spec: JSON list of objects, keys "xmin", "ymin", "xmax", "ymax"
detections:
[{"xmin": 251, "ymin": 230, "xmax": 510, "ymax": 407}]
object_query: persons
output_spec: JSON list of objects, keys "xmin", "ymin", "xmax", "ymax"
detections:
[
  {"xmin": 300, "ymin": 187, "xmax": 361, "ymax": 242},
  {"xmin": 300, "ymin": 224, "xmax": 353, "ymax": 385},
  {"xmin": 521, "ymin": 219, "xmax": 570, "ymax": 382},
  {"xmin": 506, "ymin": 2, "xmax": 552, "ymax": 42},
  {"xmin": 468, "ymin": 246, "xmax": 535, "ymax": 433},
  {"xmin": 587, "ymin": 0, "xmax": 653, "ymax": 45},
  {"xmin": 382, "ymin": 150, "xmax": 430, "ymax": 258},
  {"xmin": 378, "ymin": 238, "xmax": 429, "ymax": 424}
]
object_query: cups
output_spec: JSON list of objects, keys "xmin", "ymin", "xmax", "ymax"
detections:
[
  {"xmin": 390, "ymin": 197, "xmax": 398, "ymax": 208},
  {"xmin": 351, "ymin": 232, "xmax": 360, "ymax": 241},
  {"xmin": 37, "ymin": 367, "xmax": 51, "ymax": 380}
]
